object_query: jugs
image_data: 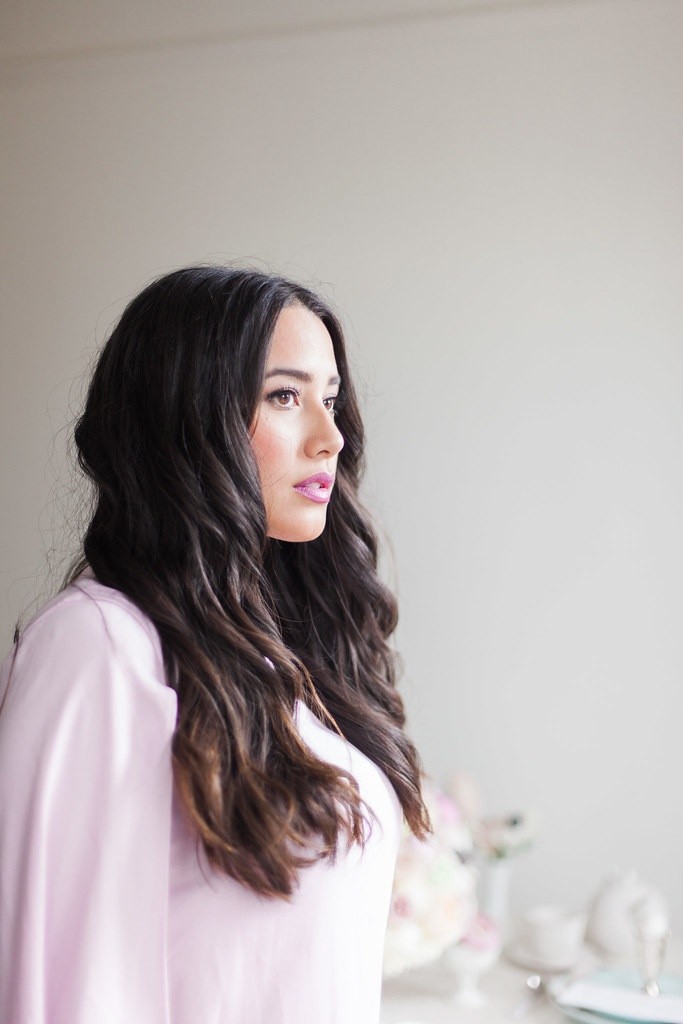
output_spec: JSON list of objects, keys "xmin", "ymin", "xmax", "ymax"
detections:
[{"xmin": 589, "ymin": 870, "xmax": 670, "ymax": 991}]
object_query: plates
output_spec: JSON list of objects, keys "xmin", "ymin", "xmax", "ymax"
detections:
[{"xmin": 547, "ymin": 977, "xmax": 683, "ymax": 1024}]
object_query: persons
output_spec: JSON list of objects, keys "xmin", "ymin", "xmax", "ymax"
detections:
[{"xmin": 0, "ymin": 262, "xmax": 435, "ymax": 1024}]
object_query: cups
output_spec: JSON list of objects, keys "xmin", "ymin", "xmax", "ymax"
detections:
[{"xmin": 525, "ymin": 908, "xmax": 586, "ymax": 966}]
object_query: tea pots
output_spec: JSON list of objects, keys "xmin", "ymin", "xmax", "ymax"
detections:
[{"xmin": 389, "ymin": 778, "xmax": 521, "ymax": 988}]
